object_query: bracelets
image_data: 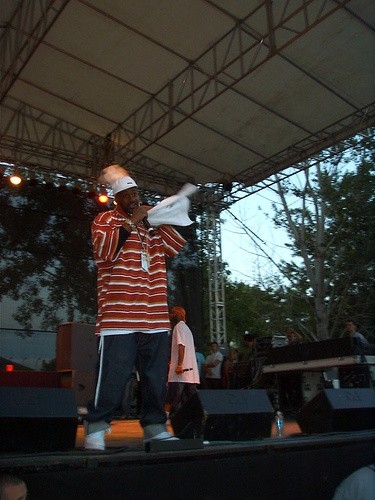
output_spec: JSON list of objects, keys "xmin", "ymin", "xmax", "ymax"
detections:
[{"xmin": 124, "ymin": 219, "xmax": 137, "ymax": 230}]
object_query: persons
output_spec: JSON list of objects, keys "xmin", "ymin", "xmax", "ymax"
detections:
[
  {"xmin": 333, "ymin": 463, "xmax": 375, "ymax": 500},
  {"xmin": 164, "ymin": 306, "xmax": 201, "ymax": 424},
  {"xmin": 194, "ymin": 322, "xmax": 370, "ymax": 390},
  {"xmin": 0, "ymin": 474, "xmax": 27, "ymax": 500},
  {"xmin": 83, "ymin": 177, "xmax": 187, "ymax": 451}
]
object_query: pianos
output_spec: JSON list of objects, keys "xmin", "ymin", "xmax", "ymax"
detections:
[{"xmin": 262, "ymin": 338, "xmax": 375, "ymax": 373}]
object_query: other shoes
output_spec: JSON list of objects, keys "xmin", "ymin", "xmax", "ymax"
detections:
[
  {"xmin": 143, "ymin": 431, "xmax": 180, "ymax": 442},
  {"xmin": 84, "ymin": 430, "xmax": 105, "ymax": 451}
]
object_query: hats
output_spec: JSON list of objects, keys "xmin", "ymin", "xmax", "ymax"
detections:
[
  {"xmin": 172, "ymin": 306, "xmax": 187, "ymax": 323},
  {"xmin": 112, "ymin": 175, "xmax": 139, "ymax": 195},
  {"xmin": 244, "ymin": 333, "xmax": 258, "ymax": 340}
]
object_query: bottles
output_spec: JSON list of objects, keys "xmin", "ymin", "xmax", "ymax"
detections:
[{"xmin": 275, "ymin": 411, "xmax": 284, "ymax": 439}]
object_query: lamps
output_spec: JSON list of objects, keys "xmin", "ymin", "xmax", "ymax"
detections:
[
  {"xmin": 72, "ymin": 181, "xmax": 82, "ymax": 195},
  {"xmin": 28, "ymin": 170, "xmax": 37, "ymax": 186},
  {"xmin": 45, "ymin": 174, "xmax": 53, "ymax": 189},
  {"xmin": 0, "ymin": 165, "xmax": 5, "ymax": 179},
  {"xmin": 98, "ymin": 193, "xmax": 108, "ymax": 203},
  {"xmin": 222, "ymin": 174, "xmax": 234, "ymax": 193},
  {"xmin": 9, "ymin": 169, "xmax": 22, "ymax": 185},
  {"xmin": 57, "ymin": 178, "xmax": 67, "ymax": 191}
]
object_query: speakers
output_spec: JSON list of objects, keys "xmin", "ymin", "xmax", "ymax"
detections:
[
  {"xmin": 55, "ymin": 324, "xmax": 99, "ymax": 407},
  {"xmin": 169, "ymin": 389, "xmax": 275, "ymax": 442},
  {"xmin": 293, "ymin": 387, "xmax": 375, "ymax": 436},
  {"xmin": 0, "ymin": 385, "xmax": 79, "ymax": 453}
]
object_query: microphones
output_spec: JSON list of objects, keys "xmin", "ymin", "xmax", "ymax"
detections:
[{"xmin": 142, "ymin": 217, "xmax": 155, "ymax": 237}]
